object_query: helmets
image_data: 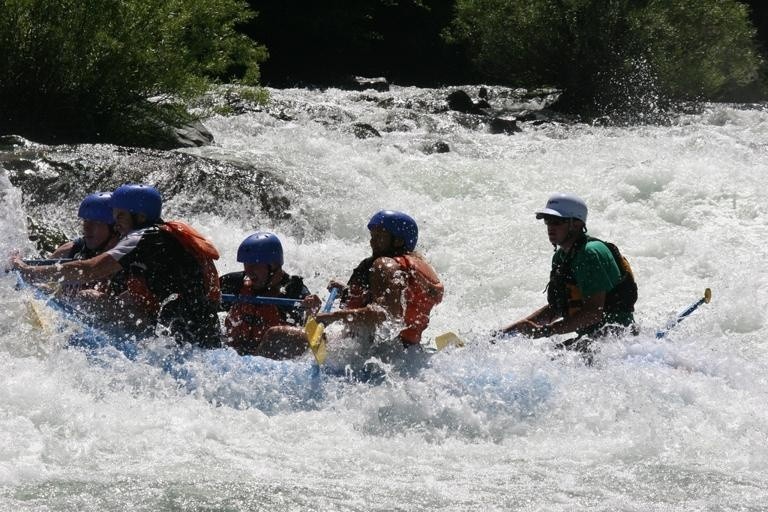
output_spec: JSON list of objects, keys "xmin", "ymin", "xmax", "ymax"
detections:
[
  {"xmin": 108, "ymin": 185, "xmax": 162, "ymax": 220},
  {"xmin": 367, "ymin": 210, "xmax": 418, "ymax": 251},
  {"xmin": 237, "ymin": 233, "xmax": 283, "ymax": 265},
  {"xmin": 78, "ymin": 192, "xmax": 116, "ymax": 224},
  {"xmin": 535, "ymin": 193, "xmax": 587, "ymax": 225}
]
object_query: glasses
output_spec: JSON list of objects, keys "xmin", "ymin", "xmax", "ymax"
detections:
[{"xmin": 543, "ymin": 215, "xmax": 576, "ymax": 226}]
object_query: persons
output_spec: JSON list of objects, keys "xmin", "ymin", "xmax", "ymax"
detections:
[
  {"xmin": 490, "ymin": 193, "xmax": 639, "ymax": 366},
  {"xmin": 47, "ymin": 191, "xmax": 127, "ymax": 320},
  {"xmin": 13, "ymin": 184, "xmax": 220, "ymax": 349},
  {"xmin": 219, "ymin": 231, "xmax": 321, "ymax": 362},
  {"xmin": 253, "ymin": 210, "xmax": 444, "ymax": 360}
]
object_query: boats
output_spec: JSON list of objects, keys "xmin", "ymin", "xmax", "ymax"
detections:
[{"xmin": 6, "ymin": 268, "xmax": 709, "ymax": 423}]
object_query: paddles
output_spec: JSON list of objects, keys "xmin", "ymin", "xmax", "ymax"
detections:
[
  {"xmin": 436, "ymin": 322, "xmax": 497, "ymax": 356},
  {"xmin": 25, "ymin": 282, "xmax": 61, "ymax": 334},
  {"xmin": 307, "ymin": 286, "xmax": 338, "ymax": 365}
]
